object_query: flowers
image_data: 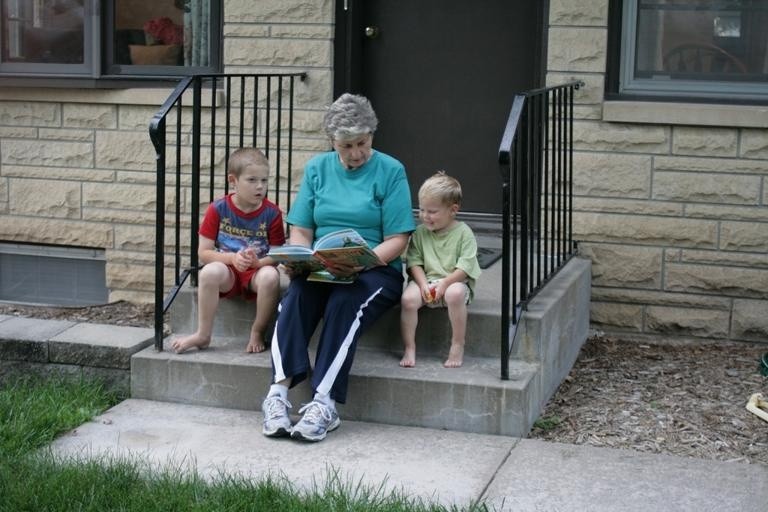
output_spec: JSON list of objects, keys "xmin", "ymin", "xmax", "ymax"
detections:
[{"xmin": 142, "ymin": 13, "xmax": 186, "ymax": 45}]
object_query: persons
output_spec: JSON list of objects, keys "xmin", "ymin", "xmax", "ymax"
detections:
[
  {"xmin": 261, "ymin": 90, "xmax": 415, "ymax": 445},
  {"xmin": 170, "ymin": 148, "xmax": 284, "ymax": 355},
  {"xmin": 399, "ymin": 172, "xmax": 483, "ymax": 369}
]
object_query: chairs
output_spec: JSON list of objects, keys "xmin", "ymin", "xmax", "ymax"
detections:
[{"xmin": 660, "ymin": 37, "xmax": 748, "ymax": 80}]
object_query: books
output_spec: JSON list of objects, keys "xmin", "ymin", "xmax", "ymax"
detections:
[
  {"xmin": 306, "ymin": 267, "xmax": 354, "ymax": 286},
  {"xmin": 266, "ymin": 228, "xmax": 387, "ymax": 279}
]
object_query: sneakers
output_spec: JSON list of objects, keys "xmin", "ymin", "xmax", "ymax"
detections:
[
  {"xmin": 290, "ymin": 399, "xmax": 341, "ymax": 443},
  {"xmin": 262, "ymin": 391, "xmax": 293, "ymax": 436}
]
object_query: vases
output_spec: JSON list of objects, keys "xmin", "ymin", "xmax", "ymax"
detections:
[{"xmin": 125, "ymin": 45, "xmax": 182, "ymax": 67}]
